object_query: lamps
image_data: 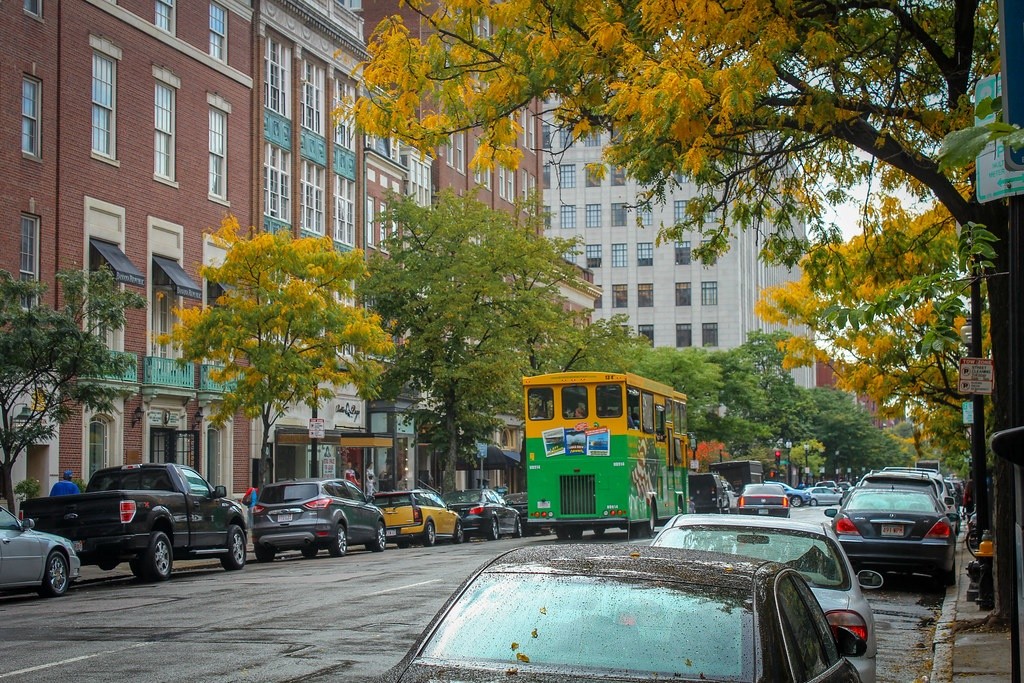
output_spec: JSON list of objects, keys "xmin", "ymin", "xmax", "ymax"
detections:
[
  {"xmin": 191, "ymin": 411, "xmax": 204, "ymax": 431},
  {"xmin": 132, "ymin": 407, "xmax": 146, "ymax": 429}
]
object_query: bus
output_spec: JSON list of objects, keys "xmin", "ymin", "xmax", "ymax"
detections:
[{"xmin": 521, "ymin": 370, "xmax": 699, "ymax": 540}]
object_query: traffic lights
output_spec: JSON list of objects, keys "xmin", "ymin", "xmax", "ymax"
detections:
[
  {"xmin": 769, "ymin": 471, "xmax": 775, "ymax": 478},
  {"xmin": 774, "ymin": 450, "xmax": 781, "ymax": 467}
]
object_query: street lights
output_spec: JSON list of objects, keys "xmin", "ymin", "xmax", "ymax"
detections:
[
  {"xmin": 804, "ymin": 443, "xmax": 810, "ymax": 488},
  {"xmin": 785, "ymin": 439, "xmax": 793, "ymax": 487},
  {"xmin": 311, "ymin": 361, "xmax": 348, "ymax": 479},
  {"xmin": 835, "ymin": 450, "xmax": 840, "ymax": 482}
]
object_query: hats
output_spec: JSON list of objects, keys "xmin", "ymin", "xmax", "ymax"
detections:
[{"xmin": 63, "ymin": 471, "xmax": 73, "ymax": 478}]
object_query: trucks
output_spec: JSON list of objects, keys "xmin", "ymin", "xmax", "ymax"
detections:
[{"xmin": 709, "ymin": 459, "xmax": 766, "ymax": 493}]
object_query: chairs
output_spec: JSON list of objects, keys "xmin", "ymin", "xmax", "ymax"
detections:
[
  {"xmin": 859, "ymin": 501, "xmax": 874, "ymax": 508},
  {"xmin": 909, "ymin": 503, "xmax": 925, "ymax": 510}
]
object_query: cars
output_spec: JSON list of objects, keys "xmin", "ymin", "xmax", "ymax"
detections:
[
  {"xmin": 501, "ymin": 492, "xmax": 556, "ymax": 537},
  {"xmin": 649, "ymin": 512, "xmax": 886, "ymax": 683},
  {"xmin": 736, "ymin": 483, "xmax": 793, "ymax": 518},
  {"xmin": 815, "ymin": 458, "xmax": 966, "ymax": 536},
  {"xmin": 822, "ymin": 483, "xmax": 961, "ymax": 588},
  {"xmin": 373, "ymin": 538, "xmax": 868, "ymax": 683},
  {"xmin": 0, "ymin": 505, "xmax": 82, "ymax": 599},
  {"xmin": 805, "ymin": 486, "xmax": 845, "ymax": 508},
  {"xmin": 440, "ymin": 487, "xmax": 523, "ymax": 543},
  {"xmin": 363, "ymin": 487, "xmax": 465, "ymax": 550}
]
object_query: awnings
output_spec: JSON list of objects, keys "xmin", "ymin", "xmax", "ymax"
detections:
[
  {"xmin": 219, "ymin": 283, "xmax": 236, "ymax": 293},
  {"xmin": 153, "ymin": 255, "xmax": 202, "ymax": 300},
  {"xmin": 89, "ymin": 239, "xmax": 145, "ymax": 286}
]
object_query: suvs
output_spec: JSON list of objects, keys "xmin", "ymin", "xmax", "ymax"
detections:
[
  {"xmin": 721, "ymin": 480, "xmax": 739, "ymax": 514},
  {"xmin": 251, "ymin": 477, "xmax": 387, "ymax": 563},
  {"xmin": 764, "ymin": 480, "xmax": 811, "ymax": 508}
]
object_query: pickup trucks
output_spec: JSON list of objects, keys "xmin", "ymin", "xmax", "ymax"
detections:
[{"xmin": 16, "ymin": 462, "xmax": 250, "ymax": 583}]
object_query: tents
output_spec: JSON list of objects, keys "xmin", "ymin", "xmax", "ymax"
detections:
[{"xmin": 431, "ymin": 444, "xmax": 520, "ymax": 489}]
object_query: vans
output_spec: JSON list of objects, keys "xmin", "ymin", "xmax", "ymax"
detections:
[{"xmin": 687, "ymin": 472, "xmax": 730, "ymax": 515}]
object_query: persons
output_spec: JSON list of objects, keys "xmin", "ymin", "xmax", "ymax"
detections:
[
  {"xmin": 962, "ymin": 479, "xmax": 974, "ymax": 530},
  {"xmin": 366, "ymin": 461, "xmax": 376, "ymax": 488},
  {"xmin": 379, "ymin": 464, "xmax": 393, "ymax": 492},
  {"xmin": 632, "ymin": 412, "xmax": 639, "ymax": 420},
  {"xmin": 797, "ymin": 480, "xmax": 805, "ymax": 489},
  {"xmin": 502, "ymin": 481, "xmax": 507, "ymax": 487},
  {"xmin": 617, "ymin": 407, "xmax": 639, "ymax": 429},
  {"xmin": 366, "ymin": 476, "xmax": 378, "ymax": 499},
  {"xmin": 575, "ymin": 408, "xmax": 585, "ymax": 417},
  {"xmin": 49, "ymin": 469, "xmax": 80, "ymax": 497},
  {"xmin": 533, "ymin": 403, "xmax": 549, "ymax": 419},
  {"xmin": 344, "ymin": 461, "xmax": 360, "ymax": 496}
]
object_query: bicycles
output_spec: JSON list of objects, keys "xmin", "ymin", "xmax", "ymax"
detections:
[{"xmin": 961, "ymin": 504, "xmax": 981, "ymax": 560}]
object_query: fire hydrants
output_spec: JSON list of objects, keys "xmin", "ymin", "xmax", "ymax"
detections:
[{"xmin": 974, "ymin": 528, "xmax": 995, "ymax": 609}]
